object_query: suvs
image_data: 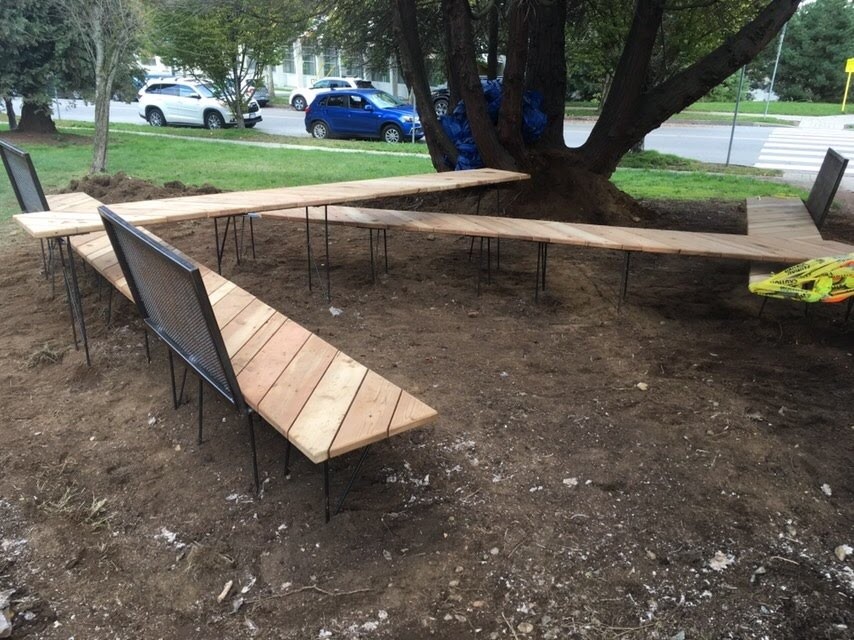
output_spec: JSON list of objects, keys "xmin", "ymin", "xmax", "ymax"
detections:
[
  {"xmin": 136, "ymin": 71, "xmax": 270, "ymax": 131},
  {"xmin": 432, "ymin": 74, "xmax": 504, "ymax": 117}
]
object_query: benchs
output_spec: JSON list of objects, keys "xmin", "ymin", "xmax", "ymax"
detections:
[{"xmin": 0, "ymin": 169, "xmax": 854, "ymax": 523}]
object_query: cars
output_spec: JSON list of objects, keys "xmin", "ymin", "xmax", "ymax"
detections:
[
  {"xmin": 304, "ymin": 89, "xmax": 425, "ymax": 145},
  {"xmin": 288, "ymin": 77, "xmax": 376, "ymax": 111}
]
